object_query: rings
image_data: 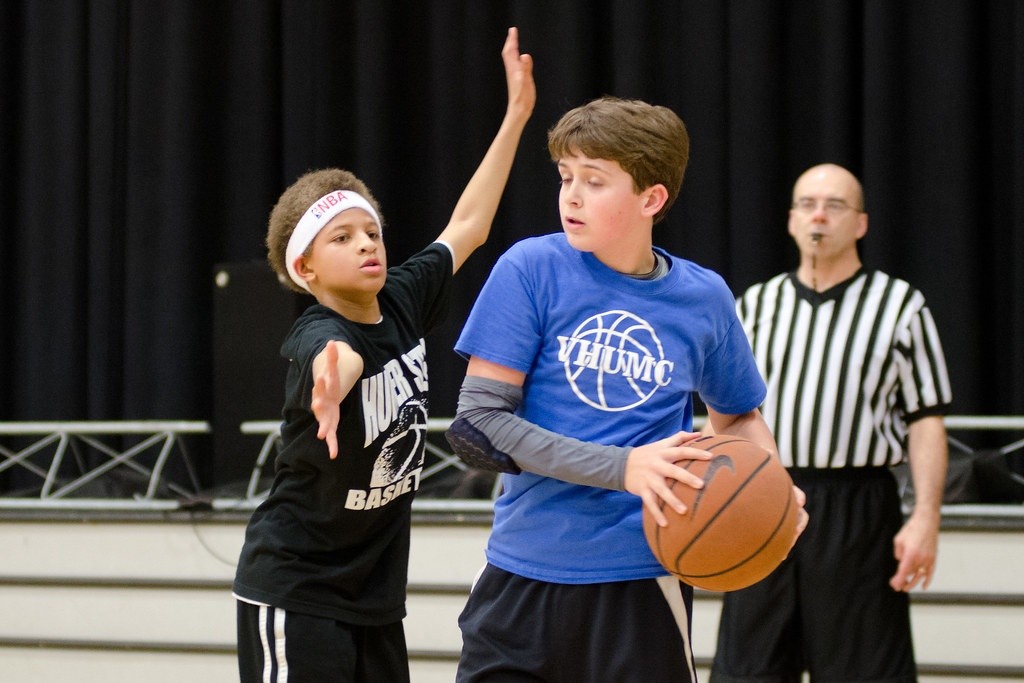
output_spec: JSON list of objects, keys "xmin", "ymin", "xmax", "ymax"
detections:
[{"xmin": 916, "ymin": 568, "xmax": 924, "ymax": 574}]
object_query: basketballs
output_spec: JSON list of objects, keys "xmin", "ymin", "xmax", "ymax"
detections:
[{"xmin": 639, "ymin": 433, "xmax": 801, "ymax": 595}]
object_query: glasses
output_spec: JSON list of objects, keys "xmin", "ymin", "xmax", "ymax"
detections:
[{"xmin": 792, "ymin": 200, "xmax": 861, "ymax": 215}]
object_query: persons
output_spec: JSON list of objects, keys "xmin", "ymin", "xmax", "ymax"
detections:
[
  {"xmin": 707, "ymin": 164, "xmax": 953, "ymax": 683},
  {"xmin": 444, "ymin": 98, "xmax": 810, "ymax": 683},
  {"xmin": 232, "ymin": 26, "xmax": 535, "ymax": 683}
]
